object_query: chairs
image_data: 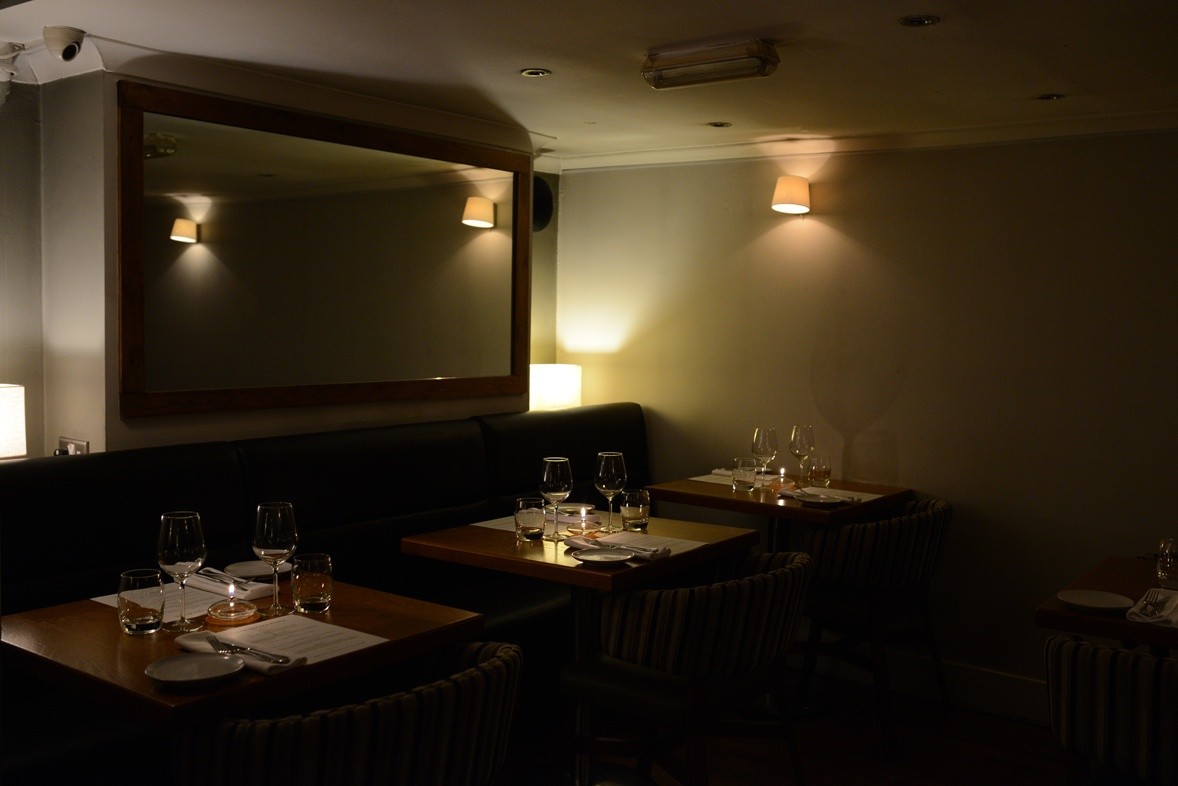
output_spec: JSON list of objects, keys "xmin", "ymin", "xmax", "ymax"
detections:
[
  {"xmin": 170, "ymin": 641, "xmax": 522, "ymax": 786},
  {"xmin": 565, "ymin": 548, "xmax": 814, "ymax": 784},
  {"xmin": 780, "ymin": 488, "xmax": 954, "ymax": 765},
  {"xmin": 1043, "ymin": 634, "xmax": 1177, "ymax": 786}
]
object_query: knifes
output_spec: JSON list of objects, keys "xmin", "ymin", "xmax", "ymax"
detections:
[
  {"xmin": 220, "ymin": 642, "xmax": 288, "ymax": 663},
  {"xmin": 1152, "ymin": 597, "xmax": 1170, "ymax": 615},
  {"xmin": 583, "ymin": 537, "xmax": 658, "ymax": 552},
  {"xmin": 195, "ymin": 570, "xmax": 250, "ymax": 592}
]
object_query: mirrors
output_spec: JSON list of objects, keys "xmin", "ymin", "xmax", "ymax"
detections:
[{"xmin": 114, "ymin": 74, "xmax": 531, "ymax": 426}]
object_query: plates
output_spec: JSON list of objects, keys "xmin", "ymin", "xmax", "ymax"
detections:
[
  {"xmin": 225, "ymin": 560, "xmax": 290, "ymax": 577},
  {"xmin": 794, "ymin": 494, "xmax": 841, "ymax": 502},
  {"xmin": 144, "ymin": 652, "xmax": 244, "ymax": 680},
  {"xmin": 544, "ymin": 502, "xmax": 596, "ymax": 513},
  {"xmin": 1056, "ymin": 589, "xmax": 1135, "ymax": 609},
  {"xmin": 740, "ymin": 467, "xmax": 771, "ymax": 472},
  {"xmin": 571, "ymin": 547, "xmax": 637, "ymax": 564}
]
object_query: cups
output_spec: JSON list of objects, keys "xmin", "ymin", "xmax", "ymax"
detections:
[
  {"xmin": 620, "ymin": 488, "xmax": 649, "ymax": 530},
  {"xmin": 514, "ymin": 498, "xmax": 546, "ymax": 541},
  {"xmin": 1155, "ymin": 538, "xmax": 1176, "ymax": 590},
  {"xmin": 733, "ymin": 458, "xmax": 757, "ymax": 491},
  {"xmin": 118, "ymin": 568, "xmax": 164, "ymax": 635},
  {"xmin": 808, "ymin": 455, "xmax": 832, "ymax": 487},
  {"xmin": 291, "ymin": 551, "xmax": 333, "ymax": 613}
]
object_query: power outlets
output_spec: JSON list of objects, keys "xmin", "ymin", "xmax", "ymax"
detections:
[{"xmin": 58, "ymin": 436, "xmax": 89, "ymax": 455}]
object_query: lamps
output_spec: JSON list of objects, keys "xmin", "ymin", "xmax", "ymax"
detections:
[
  {"xmin": 641, "ymin": 38, "xmax": 781, "ymax": 91},
  {"xmin": 770, "ymin": 175, "xmax": 810, "ymax": 214},
  {"xmin": 461, "ymin": 196, "xmax": 496, "ymax": 229},
  {"xmin": 168, "ymin": 219, "xmax": 198, "ymax": 244}
]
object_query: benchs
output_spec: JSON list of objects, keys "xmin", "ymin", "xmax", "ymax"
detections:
[{"xmin": 2, "ymin": 403, "xmax": 653, "ymax": 784}]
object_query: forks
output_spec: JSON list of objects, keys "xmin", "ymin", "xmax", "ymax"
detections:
[
  {"xmin": 203, "ymin": 568, "xmax": 258, "ymax": 585},
  {"xmin": 207, "ymin": 632, "xmax": 289, "ymax": 663},
  {"xmin": 1140, "ymin": 590, "xmax": 1159, "ymax": 614},
  {"xmin": 592, "ymin": 538, "xmax": 655, "ymax": 555}
]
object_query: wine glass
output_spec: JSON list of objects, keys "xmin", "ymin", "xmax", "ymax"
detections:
[
  {"xmin": 595, "ymin": 451, "xmax": 626, "ymax": 532},
  {"xmin": 752, "ymin": 426, "xmax": 777, "ymax": 493},
  {"xmin": 253, "ymin": 501, "xmax": 298, "ymax": 620},
  {"xmin": 159, "ymin": 510, "xmax": 207, "ymax": 633},
  {"xmin": 539, "ymin": 457, "xmax": 572, "ymax": 540},
  {"xmin": 789, "ymin": 422, "xmax": 815, "ymax": 487}
]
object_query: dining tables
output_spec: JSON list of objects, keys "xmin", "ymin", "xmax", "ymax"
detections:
[
  {"xmin": 1032, "ymin": 554, "xmax": 1178, "ymax": 662},
  {"xmin": 399, "ymin": 505, "xmax": 761, "ymax": 785},
  {"xmin": 666, "ymin": 467, "xmax": 889, "ymax": 717},
  {"xmin": 1, "ymin": 572, "xmax": 482, "ymax": 723}
]
